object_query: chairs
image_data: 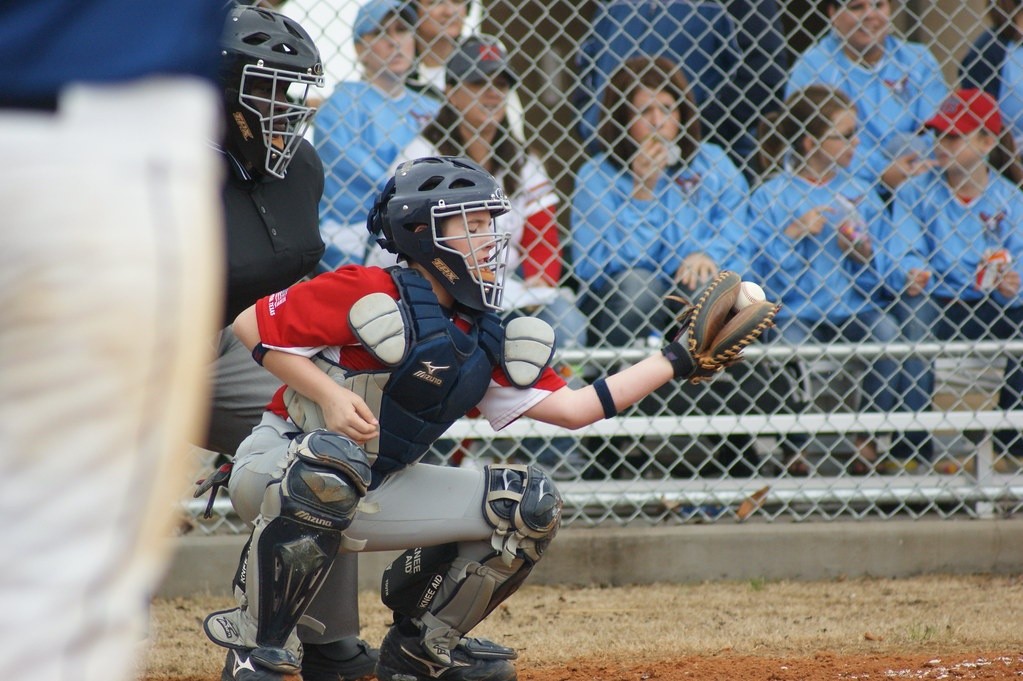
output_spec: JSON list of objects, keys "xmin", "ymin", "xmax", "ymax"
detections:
[{"xmin": 570, "ymin": 0, "xmax": 793, "ymax": 160}]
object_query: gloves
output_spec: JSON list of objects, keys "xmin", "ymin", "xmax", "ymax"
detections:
[{"xmin": 193, "ymin": 461, "xmax": 234, "ymax": 519}]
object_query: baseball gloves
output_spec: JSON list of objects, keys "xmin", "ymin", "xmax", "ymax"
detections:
[{"xmin": 662, "ymin": 269, "xmax": 781, "ymax": 383}]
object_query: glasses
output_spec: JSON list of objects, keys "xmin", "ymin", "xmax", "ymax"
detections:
[{"xmin": 824, "ymin": 127, "xmax": 858, "ymax": 141}]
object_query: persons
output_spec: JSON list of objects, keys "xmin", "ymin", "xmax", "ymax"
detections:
[
  {"xmin": 881, "ymin": 89, "xmax": 1023, "ymax": 476},
  {"xmin": 367, "ymin": 36, "xmax": 588, "ymax": 484},
  {"xmin": 747, "ymin": 83, "xmax": 903, "ymax": 479},
  {"xmin": 784, "ymin": 0, "xmax": 948, "ymax": 216},
  {"xmin": 0, "ymin": 1, "xmax": 227, "ymax": 680},
  {"xmin": 308, "ymin": 0, "xmax": 531, "ymax": 279},
  {"xmin": 958, "ymin": 0, "xmax": 1023, "ymax": 163},
  {"xmin": 185, "ymin": 6, "xmax": 326, "ymax": 457},
  {"xmin": 571, "ymin": 56, "xmax": 763, "ymax": 481},
  {"xmin": 201, "ymin": 155, "xmax": 796, "ymax": 681}
]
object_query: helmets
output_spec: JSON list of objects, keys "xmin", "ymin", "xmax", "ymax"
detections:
[
  {"xmin": 218, "ymin": 5, "xmax": 325, "ymax": 179},
  {"xmin": 367, "ymin": 156, "xmax": 512, "ymax": 316}
]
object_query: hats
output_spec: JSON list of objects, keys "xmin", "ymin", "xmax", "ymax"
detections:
[
  {"xmin": 446, "ymin": 33, "xmax": 519, "ymax": 87},
  {"xmin": 919, "ymin": 88, "xmax": 1002, "ymax": 138},
  {"xmin": 353, "ymin": 0, "xmax": 416, "ymax": 42}
]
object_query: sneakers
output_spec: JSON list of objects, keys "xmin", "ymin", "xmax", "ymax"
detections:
[
  {"xmin": 220, "ymin": 646, "xmax": 303, "ymax": 681},
  {"xmin": 374, "ymin": 625, "xmax": 517, "ymax": 681},
  {"xmin": 301, "ymin": 639, "xmax": 381, "ymax": 681}
]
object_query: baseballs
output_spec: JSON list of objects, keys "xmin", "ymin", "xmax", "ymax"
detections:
[{"xmin": 735, "ymin": 281, "xmax": 766, "ymax": 311}]
